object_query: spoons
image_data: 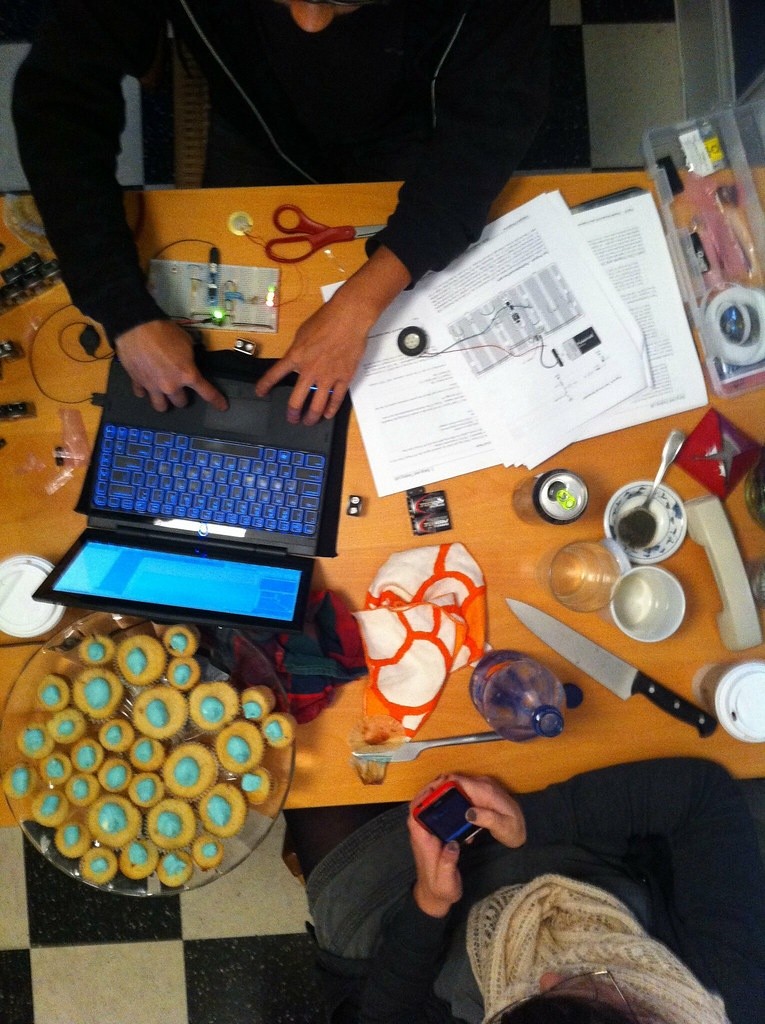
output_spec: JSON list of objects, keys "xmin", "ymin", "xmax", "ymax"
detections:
[{"xmin": 618, "ymin": 429, "xmax": 685, "ymax": 547}]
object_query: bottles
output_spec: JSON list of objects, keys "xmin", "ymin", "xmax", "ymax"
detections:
[{"xmin": 470, "ymin": 650, "xmax": 566, "ymax": 742}]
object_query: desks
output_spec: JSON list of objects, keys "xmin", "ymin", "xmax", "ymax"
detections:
[{"xmin": 0, "ymin": 166, "xmax": 765, "ymax": 830}]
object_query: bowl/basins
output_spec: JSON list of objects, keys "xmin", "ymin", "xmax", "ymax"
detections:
[{"xmin": 603, "ymin": 482, "xmax": 687, "ymax": 565}]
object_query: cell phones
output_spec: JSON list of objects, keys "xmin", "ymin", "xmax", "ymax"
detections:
[{"xmin": 413, "ymin": 781, "xmax": 485, "ymax": 846}]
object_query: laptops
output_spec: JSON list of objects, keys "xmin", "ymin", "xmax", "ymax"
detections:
[{"xmin": 32, "ymin": 356, "xmax": 339, "ymax": 633}]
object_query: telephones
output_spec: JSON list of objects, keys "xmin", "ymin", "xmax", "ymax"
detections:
[{"xmin": 684, "ymin": 493, "xmax": 762, "ymax": 654}]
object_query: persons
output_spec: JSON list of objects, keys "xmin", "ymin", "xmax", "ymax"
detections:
[
  {"xmin": 8, "ymin": 0, "xmax": 556, "ymax": 426},
  {"xmin": 285, "ymin": 757, "xmax": 765, "ymax": 1024}
]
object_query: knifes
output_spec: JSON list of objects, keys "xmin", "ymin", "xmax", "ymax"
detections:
[
  {"xmin": 506, "ymin": 598, "xmax": 718, "ymax": 736},
  {"xmin": 351, "ymin": 730, "xmax": 506, "ymax": 761}
]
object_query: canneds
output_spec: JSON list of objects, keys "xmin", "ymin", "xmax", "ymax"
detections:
[{"xmin": 512, "ymin": 468, "xmax": 588, "ymax": 527}]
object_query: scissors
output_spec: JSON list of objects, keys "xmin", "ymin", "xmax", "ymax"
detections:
[{"xmin": 263, "ymin": 202, "xmax": 389, "ymax": 264}]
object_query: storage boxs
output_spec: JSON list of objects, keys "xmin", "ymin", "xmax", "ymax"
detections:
[{"xmin": 640, "ymin": 96, "xmax": 765, "ymax": 398}]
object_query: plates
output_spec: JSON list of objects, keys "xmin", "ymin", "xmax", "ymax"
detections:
[{"xmin": 1, "ymin": 612, "xmax": 294, "ymax": 897}]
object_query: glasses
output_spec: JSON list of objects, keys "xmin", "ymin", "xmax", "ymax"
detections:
[{"xmin": 484, "ymin": 967, "xmax": 639, "ymax": 1024}]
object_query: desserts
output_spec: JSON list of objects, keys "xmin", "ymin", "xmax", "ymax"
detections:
[{"xmin": 2, "ymin": 625, "xmax": 297, "ymax": 889}]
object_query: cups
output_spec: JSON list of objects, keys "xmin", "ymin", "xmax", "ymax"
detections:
[
  {"xmin": 692, "ymin": 661, "xmax": 764, "ymax": 744},
  {"xmin": 539, "ymin": 541, "xmax": 685, "ymax": 643},
  {"xmin": 0, "ymin": 553, "xmax": 67, "ymax": 638}
]
